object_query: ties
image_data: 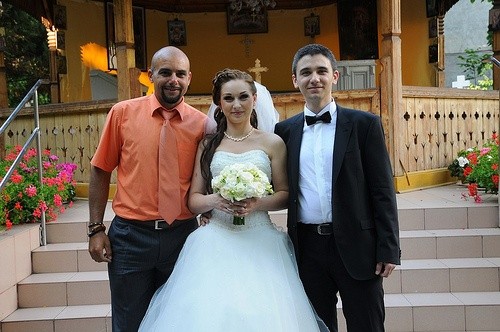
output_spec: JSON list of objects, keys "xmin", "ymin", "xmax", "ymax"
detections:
[{"xmin": 158, "ymin": 108, "xmax": 181, "ymax": 225}]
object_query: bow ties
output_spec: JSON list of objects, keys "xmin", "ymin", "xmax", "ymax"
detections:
[{"xmin": 305, "ymin": 111, "xmax": 332, "ymax": 126}]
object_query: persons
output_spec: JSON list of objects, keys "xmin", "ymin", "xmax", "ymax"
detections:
[
  {"xmin": 199, "ymin": 44, "xmax": 401, "ymax": 332},
  {"xmin": 88, "ymin": 44, "xmax": 213, "ymax": 332},
  {"xmin": 138, "ymin": 68, "xmax": 330, "ymax": 331}
]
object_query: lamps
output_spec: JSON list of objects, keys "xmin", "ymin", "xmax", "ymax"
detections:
[{"xmin": 231, "ymin": 0, "xmax": 276, "ymax": 21}]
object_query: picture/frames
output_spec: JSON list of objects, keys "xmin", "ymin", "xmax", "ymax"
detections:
[
  {"xmin": 304, "ymin": 16, "xmax": 319, "ymax": 36},
  {"xmin": 167, "ymin": 20, "xmax": 186, "ymax": 46},
  {"xmin": 227, "ymin": 8, "xmax": 268, "ymax": 34}
]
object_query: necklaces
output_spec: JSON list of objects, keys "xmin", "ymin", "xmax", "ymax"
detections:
[{"xmin": 224, "ymin": 127, "xmax": 255, "ymax": 142}]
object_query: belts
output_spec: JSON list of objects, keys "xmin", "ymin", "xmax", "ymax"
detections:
[
  {"xmin": 120, "ymin": 217, "xmax": 197, "ymax": 231},
  {"xmin": 299, "ymin": 223, "xmax": 335, "ymax": 236}
]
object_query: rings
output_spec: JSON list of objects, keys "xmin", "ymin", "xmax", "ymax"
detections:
[{"xmin": 200, "ymin": 215, "xmax": 202, "ymax": 221}]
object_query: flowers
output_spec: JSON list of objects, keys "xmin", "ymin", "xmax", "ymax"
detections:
[{"xmin": 212, "ymin": 164, "xmax": 274, "ymax": 225}]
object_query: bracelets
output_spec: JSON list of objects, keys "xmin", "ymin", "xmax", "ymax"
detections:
[{"xmin": 87, "ymin": 222, "xmax": 106, "ymax": 237}]
